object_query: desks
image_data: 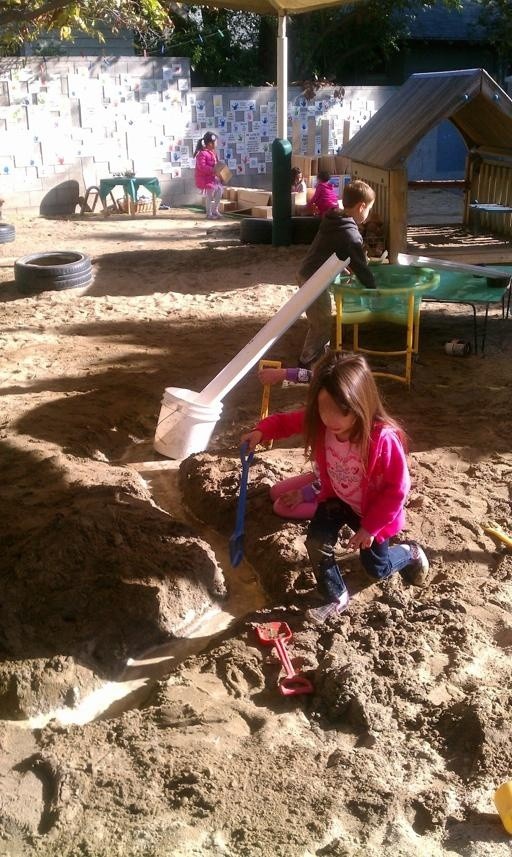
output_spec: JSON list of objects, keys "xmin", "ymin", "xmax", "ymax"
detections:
[
  {"xmin": 421, "ymin": 266, "xmax": 512, "ymax": 359},
  {"xmin": 99, "ymin": 177, "xmax": 161, "ymax": 216},
  {"xmin": 468, "ymin": 203, "xmax": 512, "ymax": 245}
]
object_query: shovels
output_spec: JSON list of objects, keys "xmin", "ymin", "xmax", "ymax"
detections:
[
  {"xmin": 256, "ymin": 622, "xmax": 313, "ymax": 697},
  {"xmin": 258, "ymin": 360, "xmax": 280, "ymax": 447},
  {"xmin": 229, "ymin": 443, "xmax": 253, "ymax": 568}
]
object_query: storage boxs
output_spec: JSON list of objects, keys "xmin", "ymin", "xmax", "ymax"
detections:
[
  {"xmin": 201, "ymin": 185, "xmax": 316, "ymax": 219},
  {"xmin": 214, "ymin": 161, "xmax": 233, "ymax": 185}
]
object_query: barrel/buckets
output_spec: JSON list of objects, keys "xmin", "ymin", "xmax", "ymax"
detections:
[{"xmin": 152, "ymin": 388, "xmax": 224, "ymax": 460}]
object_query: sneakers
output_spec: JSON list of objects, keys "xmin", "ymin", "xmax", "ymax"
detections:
[
  {"xmin": 297, "ymin": 351, "xmax": 325, "ymax": 371},
  {"xmin": 206, "ymin": 209, "xmax": 222, "ymax": 220},
  {"xmin": 406, "ymin": 540, "xmax": 428, "ymax": 586},
  {"xmin": 306, "ymin": 591, "xmax": 349, "ymax": 624}
]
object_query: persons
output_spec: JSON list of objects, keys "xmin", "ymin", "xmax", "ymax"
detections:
[
  {"xmin": 257, "ymin": 366, "xmax": 315, "ymax": 387},
  {"xmin": 290, "ymin": 166, "xmax": 306, "ymax": 192},
  {"xmin": 297, "ymin": 178, "xmax": 379, "ymax": 369},
  {"xmin": 302, "ymin": 170, "xmax": 340, "ymax": 219},
  {"xmin": 238, "ymin": 349, "xmax": 431, "ymax": 629},
  {"xmin": 193, "ymin": 130, "xmax": 226, "ymax": 219}
]
